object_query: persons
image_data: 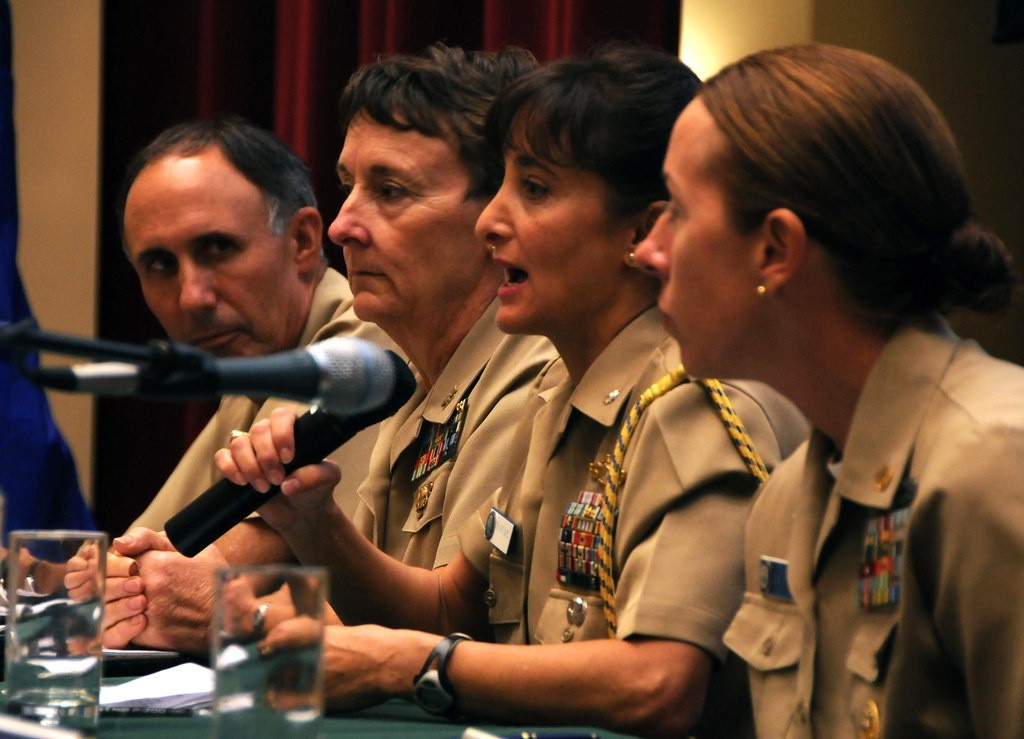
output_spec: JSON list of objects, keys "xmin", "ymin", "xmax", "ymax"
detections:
[
  {"xmin": 98, "ymin": 44, "xmax": 555, "ymax": 654},
  {"xmin": 0, "ymin": 119, "xmax": 419, "ymax": 609},
  {"xmin": 221, "ymin": 574, "xmax": 321, "ymax": 709},
  {"xmin": 635, "ymin": 41, "xmax": 1024, "ymax": 738},
  {"xmin": 214, "ymin": 46, "xmax": 813, "ymax": 732},
  {"xmin": 16, "ymin": 541, "xmax": 104, "ymax": 655}
]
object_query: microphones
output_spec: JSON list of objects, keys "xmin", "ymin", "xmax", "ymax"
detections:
[
  {"xmin": 35, "ymin": 335, "xmax": 396, "ymax": 417},
  {"xmin": 164, "ymin": 350, "xmax": 416, "ymax": 558}
]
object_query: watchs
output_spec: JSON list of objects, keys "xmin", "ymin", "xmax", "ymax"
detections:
[
  {"xmin": 413, "ymin": 634, "xmax": 472, "ymax": 717},
  {"xmin": 24, "ymin": 559, "xmax": 45, "ymax": 593},
  {"xmin": 0, "ymin": 557, "xmax": 9, "ymax": 608},
  {"xmin": 253, "ymin": 602, "xmax": 269, "ymax": 627}
]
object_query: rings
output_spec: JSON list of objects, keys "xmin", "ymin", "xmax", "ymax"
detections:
[{"xmin": 230, "ymin": 430, "xmax": 250, "ymax": 443}]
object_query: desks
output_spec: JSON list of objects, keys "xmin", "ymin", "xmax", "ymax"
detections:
[{"xmin": 0, "ymin": 598, "xmax": 641, "ymax": 739}]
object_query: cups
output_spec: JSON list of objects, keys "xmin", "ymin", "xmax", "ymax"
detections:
[
  {"xmin": 0, "ymin": 529, "xmax": 109, "ymax": 729},
  {"xmin": 211, "ymin": 563, "xmax": 330, "ymax": 722}
]
